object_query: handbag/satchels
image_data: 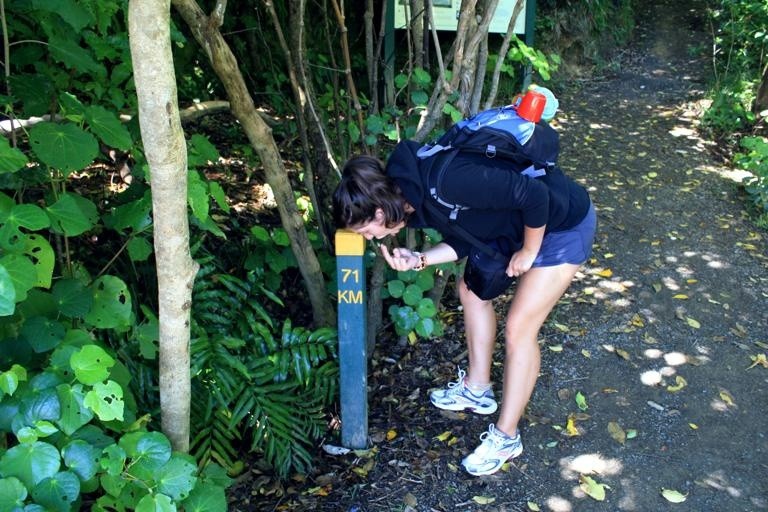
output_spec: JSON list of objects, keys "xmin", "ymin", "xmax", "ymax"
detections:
[{"xmin": 463, "ymin": 242, "xmax": 522, "ymax": 302}]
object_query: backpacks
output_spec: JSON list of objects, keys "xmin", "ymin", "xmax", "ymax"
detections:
[{"xmin": 411, "ymin": 102, "xmax": 566, "ymax": 224}]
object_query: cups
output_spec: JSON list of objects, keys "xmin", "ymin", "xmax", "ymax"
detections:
[{"xmin": 511, "ymin": 89, "xmax": 547, "ymax": 123}]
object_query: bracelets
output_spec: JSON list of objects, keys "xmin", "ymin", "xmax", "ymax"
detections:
[{"xmin": 411, "ymin": 251, "xmax": 427, "ymax": 272}]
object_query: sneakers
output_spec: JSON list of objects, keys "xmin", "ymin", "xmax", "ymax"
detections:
[
  {"xmin": 460, "ymin": 423, "xmax": 524, "ymax": 479},
  {"xmin": 432, "ymin": 365, "xmax": 499, "ymax": 417}
]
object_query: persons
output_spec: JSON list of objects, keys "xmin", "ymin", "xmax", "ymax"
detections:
[{"xmin": 331, "ymin": 140, "xmax": 597, "ymax": 477}]
